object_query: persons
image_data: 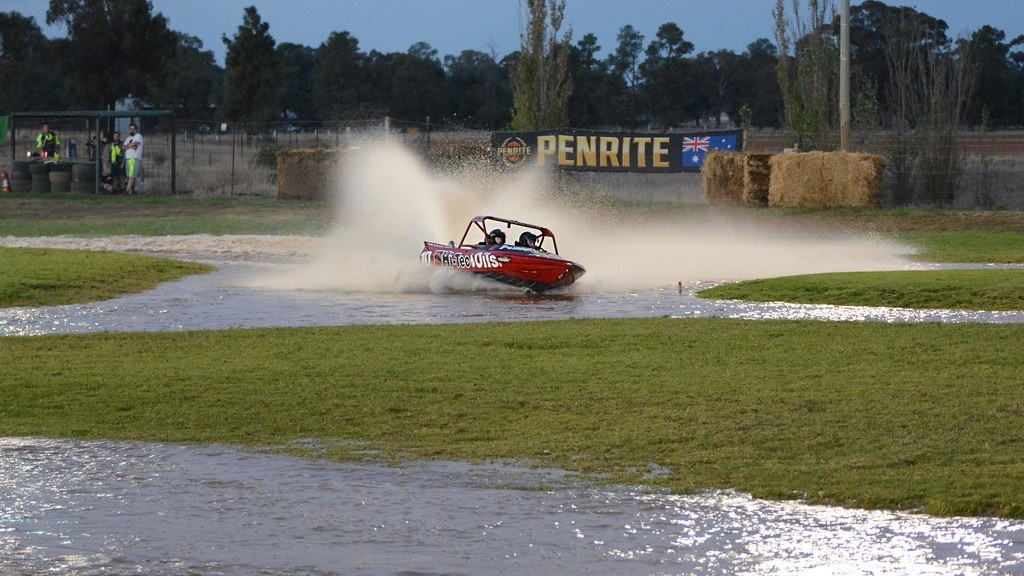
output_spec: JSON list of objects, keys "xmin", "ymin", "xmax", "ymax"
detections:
[
  {"xmin": 122, "ymin": 124, "xmax": 143, "ymax": 194},
  {"xmin": 489, "ymin": 230, "xmax": 506, "ymax": 246},
  {"xmin": 519, "ymin": 232, "xmax": 536, "ymax": 247},
  {"xmin": 93, "ymin": 135, "xmax": 104, "ymax": 145},
  {"xmin": 36, "ymin": 123, "xmax": 60, "ymax": 159},
  {"xmin": 104, "ymin": 132, "xmax": 122, "ymax": 193}
]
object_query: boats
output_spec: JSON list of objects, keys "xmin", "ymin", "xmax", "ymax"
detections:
[{"xmin": 419, "ymin": 216, "xmax": 587, "ymax": 292}]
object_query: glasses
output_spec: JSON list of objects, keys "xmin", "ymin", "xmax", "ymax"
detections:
[{"xmin": 130, "ymin": 128, "xmax": 135, "ymax": 130}]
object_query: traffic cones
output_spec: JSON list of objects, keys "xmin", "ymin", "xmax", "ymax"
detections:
[{"xmin": 2, "ymin": 173, "xmax": 11, "ymax": 192}]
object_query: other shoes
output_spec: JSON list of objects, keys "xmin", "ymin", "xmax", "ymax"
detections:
[{"xmin": 124, "ymin": 191, "xmax": 129, "ymax": 195}]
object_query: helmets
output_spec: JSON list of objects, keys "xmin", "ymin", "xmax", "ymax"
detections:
[
  {"xmin": 519, "ymin": 232, "xmax": 537, "ymax": 248},
  {"xmin": 490, "ymin": 229, "xmax": 506, "ymax": 247}
]
